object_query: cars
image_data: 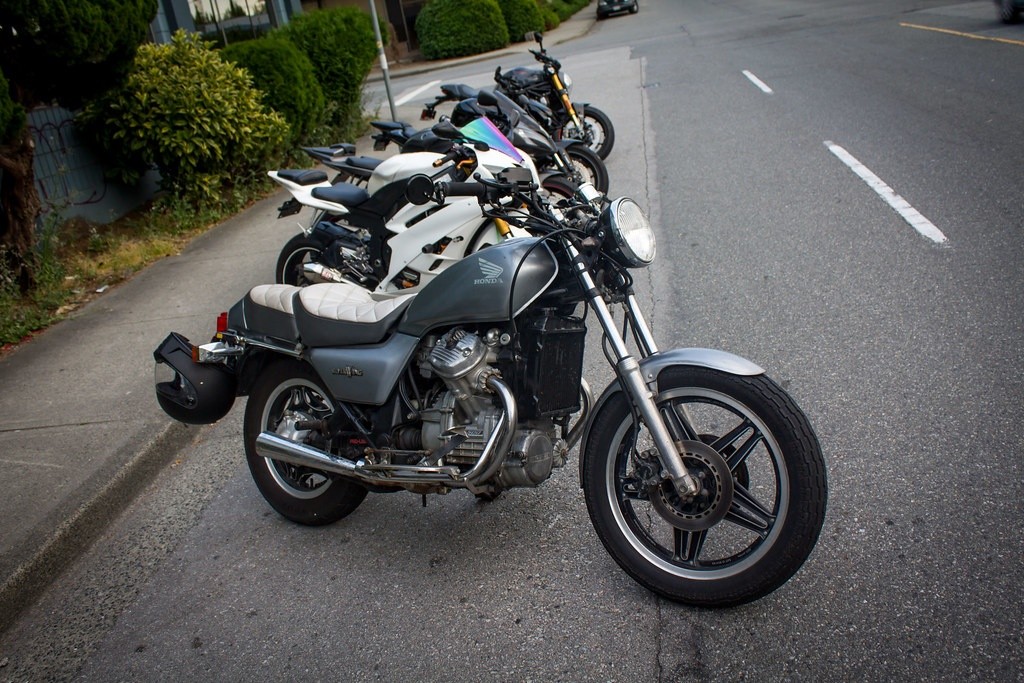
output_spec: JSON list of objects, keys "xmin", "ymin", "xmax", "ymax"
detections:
[{"xmin": 596, "ymin": 0, "xmax": 639, "ymax": 21}]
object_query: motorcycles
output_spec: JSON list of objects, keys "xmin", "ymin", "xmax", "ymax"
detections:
[
  {"xmin": 264, "ymin": 30, "xmax": 615, "ymax": 301},
  {"xmin": 155, "ymin": 168, "xmax": 830, "ymax": 610}
]
